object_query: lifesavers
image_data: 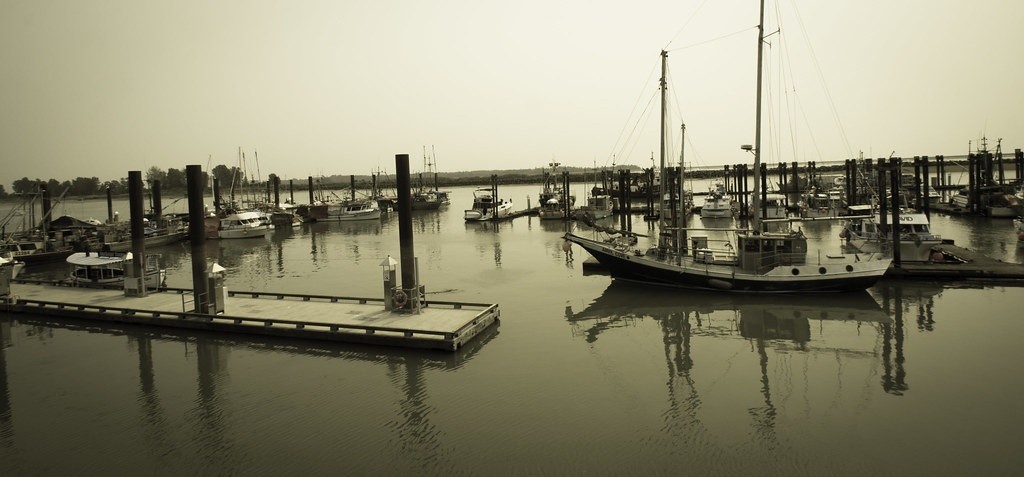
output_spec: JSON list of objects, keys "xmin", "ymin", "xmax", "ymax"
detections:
[{"xmin": 392, "ymin": 290, "xmax": 408, "ymax": 308}]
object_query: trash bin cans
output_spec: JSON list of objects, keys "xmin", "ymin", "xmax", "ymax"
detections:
[
  {"xmin": 121, "ymin": 251, "xmax": 134, "ymax": 295},
  {"xmin": 378, "ymin": 253, "xmax": 399, "ymax": 310},
  {"xmin": 203, "ymin": 262, "xmax": 227, "ymax": 315}
]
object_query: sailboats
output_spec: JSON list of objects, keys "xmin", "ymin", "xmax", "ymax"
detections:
[
  {"xmin": 0, "ymin": 144, "xmax": 450, "ymax": 262},
  {"xmin": 953, "ymin": 137, "xmax": 1015, "ymax": 218},
  {"xmin": 567, "ymin": 281, "xmax": 939, "ymax": 438},
  {"xmin": 575, "ymin": 159, "xmax": 613, "ymax": 221},
  {"xmin": 562, "ymin": 0, "xmax": 894, "ymax": 298},
  {"xmin": 654, "ymin": 124, "xmax": 693, "ymax": 219}
]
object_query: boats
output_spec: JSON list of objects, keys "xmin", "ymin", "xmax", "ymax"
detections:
[
  {"xmin": 316, "ymin": 202, "xmax": 381, "ymax": 221},
  {"xmin": 777, "ymin": 138, "xmax": 941, "ymax": 207},
  {"xmin": 538, "ymin": 162, "xmax": 576, "ymax": 219},
  {"xmin": 217, "ymin": 212, "xmax": 274, "ymax": 238},
  {"xmin": 66, "ymin": 252, "xmax": 166, "ymax": 289},
  {"xmin": 0, "ymin": 258, "xmax": 26, "ymax": 280},
  {"xmin": 849, "ymin": 193, "xmax": 941, "ymax": 262},
  {"xmin": 465, "ymin": 186, "xmax": 513, "ymax": 221},
  {"xmin": 749, "ymin": 193, "xmax": 786, "ymax": 219},
  {"xmin": 1013, "ymin": 191, "xmax": 1024, "ymax": 241},
  {"xmin": 87, "ymin": 220, "xmax": 182, "ymax": 252},
  {"xmin": 702, "ymin": 177, "xmax": 733, "ymax": 219},
  {"xmin": 616, "ymin": 170, "xmax": 660, "ymax": 198}
]
